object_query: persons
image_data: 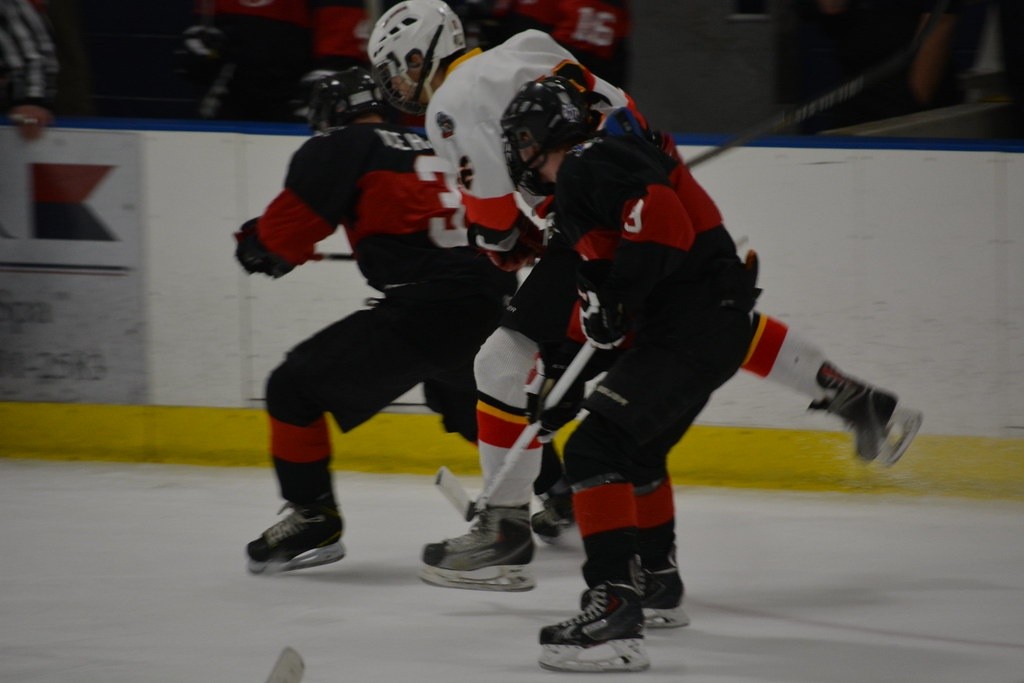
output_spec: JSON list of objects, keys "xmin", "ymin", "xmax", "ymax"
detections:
[
  {"xmin": 234, "ymin": 1, "xmax": 923, "ymax": 674},
  {"xmin": 792, "ymin": 0, "xmax": 964, "ymax": 136},
  {"xmin": 0, "ymin": 1, "xmax": 632, "ymax": 139}
]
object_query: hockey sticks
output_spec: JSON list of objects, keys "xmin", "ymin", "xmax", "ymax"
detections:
[
  {"xmin": 265, "ymin": 646, "xmax": 305, "ymax": 683},
  {"xmin": 434, "ymin": 339, "xmax": 597, "ymax": 523},
  {"xmin": 308, "ymin": 253, "xmax": 355, "ymax": 261},
  {"xmin": 511, "ymin": 68, "xmax": 880, "ymax": 231}
]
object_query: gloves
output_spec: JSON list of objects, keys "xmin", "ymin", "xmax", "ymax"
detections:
[
  {"xmin": 486, "ymin": 219, "xmax": 545, "ymax": 271},
  {"xmin": 574, "ymin": 284, "xmax": 628, "ymax": 371}
]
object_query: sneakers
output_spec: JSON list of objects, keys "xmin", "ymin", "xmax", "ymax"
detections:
[
  {"xmin": 246, "ymin": 469, "xmax": 347, "ymax": 572},
  {"xmin": 418, "ymin": 505, "xmax": 536, "ymax": 591},
  {"xmin": 807, "ymin": 361, "xmax": 924, "ymax": 469},
  {"xmin": 639, "ymin": 529, "xmax": 691, "ymax": 628},
  {"xmin": 538, "ymin": 553, "xmax": 650, "ymax": 671},
  {"xmin": 529, "ymin": 469, "xmax": 575, "ymax": 548}
]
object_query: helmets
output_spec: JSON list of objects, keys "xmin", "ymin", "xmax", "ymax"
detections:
[
  {"xmin": 366, "ymin": 1, "xmax": 465, "ymax": 74},
  {"xmin": 307, "ymin": 67, "xmax": 385, "ymax": 130},
  {"xmin": 500, "ymin": 76, "xmax": 596, "ymax": 195}
]
